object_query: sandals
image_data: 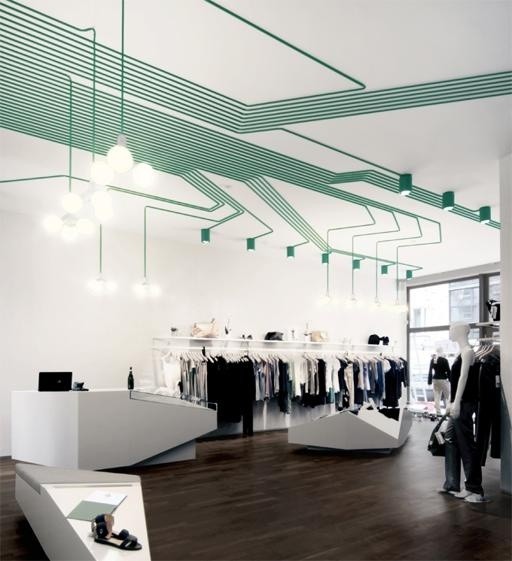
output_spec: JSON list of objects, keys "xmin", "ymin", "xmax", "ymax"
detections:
[{"xmin": 93, "ymin": 512, "xmax": 142, "ymax": 551}]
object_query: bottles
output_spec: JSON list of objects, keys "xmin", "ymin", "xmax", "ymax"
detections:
[{"xmin": 128, "ymin": 367, "xmax": 134, "ymax": 389}]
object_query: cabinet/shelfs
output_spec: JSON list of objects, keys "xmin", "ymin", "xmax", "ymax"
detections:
[
  {"xmin": 154, "ymin": 335, "xmax": 394, "ymax": 354},
  {"xmin": 12, "ymin": 463, "xmax": 151, "ymax": 561},
  {"xmin": 288, "ymin": 404, "xmax": 412, "ymax": 451}
]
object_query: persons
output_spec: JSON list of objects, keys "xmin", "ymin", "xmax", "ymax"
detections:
[
  {"xmin": 437, "ymin": 319, "xmax": 484, "ymax": 502},
  {"xmin": 427, "ymin": 347, "xmax": 452, "ymax": 418}
]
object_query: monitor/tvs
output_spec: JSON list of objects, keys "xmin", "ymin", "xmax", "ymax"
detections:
[{"xmin": 38, "ymin": 372, "xmax": 72, "ymax": 391}]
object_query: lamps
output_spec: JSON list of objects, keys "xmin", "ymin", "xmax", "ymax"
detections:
[
  {"xmin": 396, "ymin": 173, "xmax": 413, "ymax": 198},
  {"xmin": 247, "ymin": 238, "xmax": 256, "ymax": 252},
  {"xmin": 353, "ymin": 259, "xmax": 360, "ymax": 269},
  {"xmin": 405, "ymin": 270, "xmax": 413, "ymax": 279},
  {"xmin": 321, "ymin": 253, "xmax": 330, "ymax": 264},
  {"xmin": 479, "ymin": 205, "xmax": 490, "ymax": 224},
  {"xmin": 286, "ymin": 246, "xmax": 295, "ymax": 260},
  {"xmin": 381, "ymin": 265, "xmax": 388, "ymax": 275},
  {"xmin": 443, "ymin": 191, "xmax": 455, "ymax": 211},
  {"xmin": 201, "ymin": 228, "xmax": 211, "ymax": 245}
]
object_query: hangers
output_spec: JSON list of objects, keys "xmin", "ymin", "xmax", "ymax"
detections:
[
  {"xmin": 165, "ymin": 347, "xmax": 411, "ymax": 364},
  {"xmin": 475, "ymin": 335, "xmax": 500, "ymax": 361}
]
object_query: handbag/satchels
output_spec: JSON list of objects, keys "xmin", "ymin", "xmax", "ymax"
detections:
[{"xmin": 427, "ymin": 428, "xmax": 446, "ymax": 458}]
